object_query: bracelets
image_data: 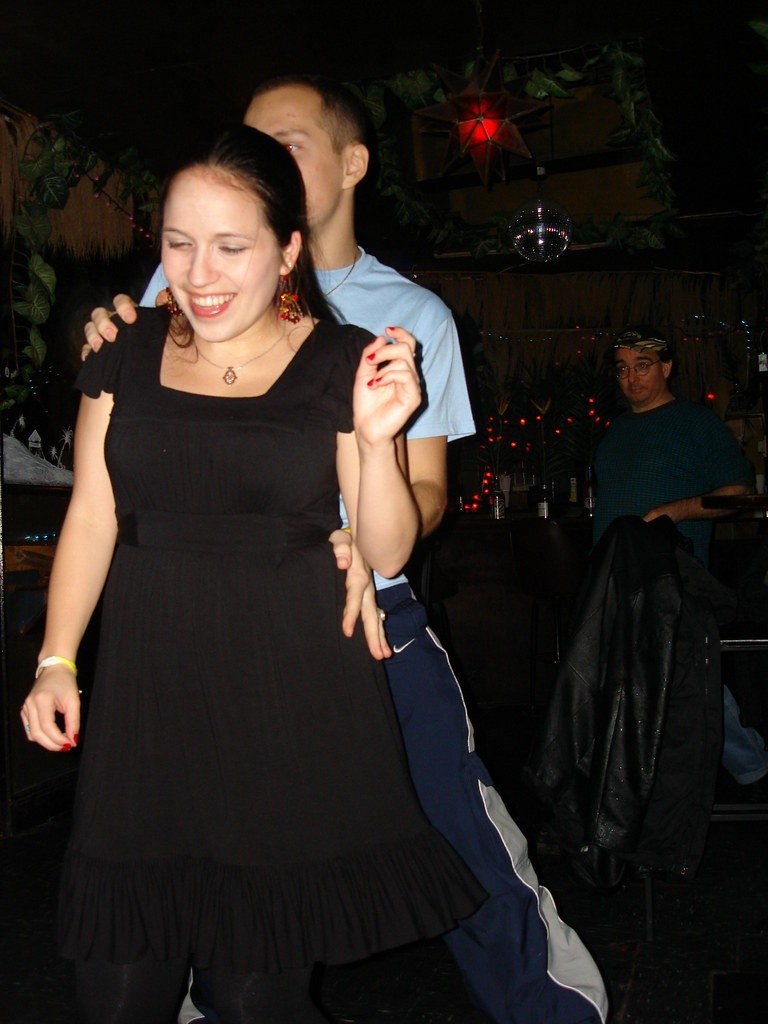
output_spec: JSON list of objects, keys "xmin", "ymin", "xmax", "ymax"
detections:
[{"xmin": 35, "ymin": 656, "xmax": 78, "ymax": 679}]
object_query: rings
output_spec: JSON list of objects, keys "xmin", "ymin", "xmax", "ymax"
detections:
[{"xmin": 25, "ymin": 724, "xmax": 30, "ymax": 731}]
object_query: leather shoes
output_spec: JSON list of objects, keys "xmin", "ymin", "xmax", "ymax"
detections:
[{"xmin": 746, "ymin": 775, "xmax": 768, "ymax": 803}]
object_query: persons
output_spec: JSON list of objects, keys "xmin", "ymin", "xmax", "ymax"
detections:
[
  {"xmin": 20, "ymin": 69, "xmax": 607, "ymax": 1024},
  {"xmin": 541, "ymin": 328, "xmax": 754, "ymax": 891},
  {"xmin": 721, "ymin": 683, "xmax": 768, "ymax": 785}
]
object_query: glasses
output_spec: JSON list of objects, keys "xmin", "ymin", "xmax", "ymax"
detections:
[{"xmin": 615, "ymin": 359, "xmax": 662, "ymax": 379}]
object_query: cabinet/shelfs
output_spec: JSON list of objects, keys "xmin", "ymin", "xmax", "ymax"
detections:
[{"xmin": 417, "ymin": 142, "xmax": 692, "ymax": 271}]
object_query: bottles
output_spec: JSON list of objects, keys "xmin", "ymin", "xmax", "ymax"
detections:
[
  {"xmin": 582, "ymin": 465, "xmax": 596, "ymax": 518},
  {"xmin": 536, "ymin": 482, "xmax": 548, "ymax": 519},
  {"xmin": 490, "ymin": 474, "xmax": 505, "ymax": 520}
]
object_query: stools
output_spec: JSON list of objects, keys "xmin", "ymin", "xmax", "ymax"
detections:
[
  {"xmin": 423, "ymin": 534, "xmax": 458, "ymax": 659},
  {"xmin": 517, "ymin": 518, "xmax": 593, "ymax": 713}
]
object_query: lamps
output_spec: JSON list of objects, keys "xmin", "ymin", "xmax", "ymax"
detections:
[{"xmin": 509, "ymin": 163, "xmax": 572, "ymax": 262}]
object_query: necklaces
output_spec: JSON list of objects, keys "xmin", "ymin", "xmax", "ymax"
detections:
[{"xmin": 194, "ymin": 321, "xmax": 287, "ymax": 384}]
